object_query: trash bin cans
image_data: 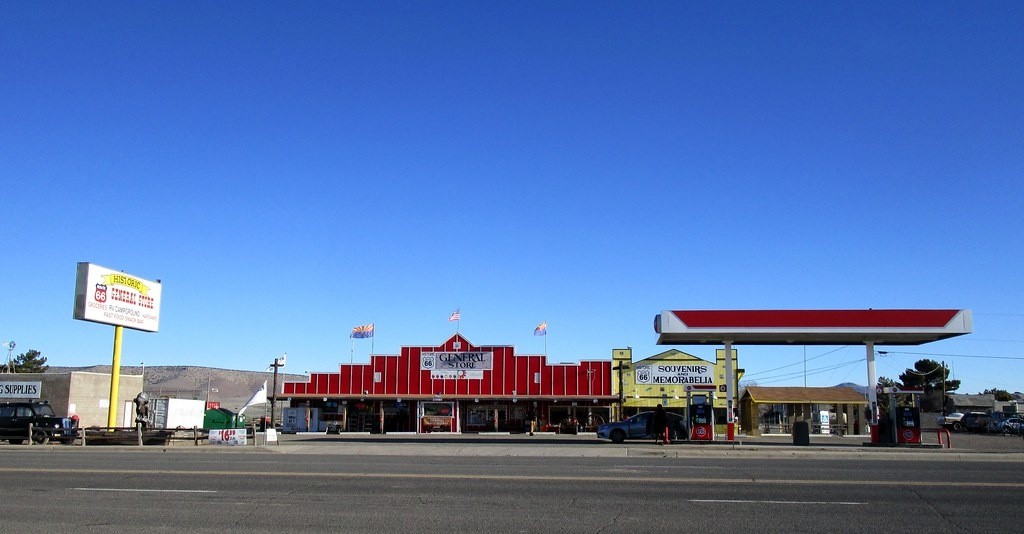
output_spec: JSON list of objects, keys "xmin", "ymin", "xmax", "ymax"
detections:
[{"xmin": 205, "ymin": 407, "xmax": 246, "ymax": 429}]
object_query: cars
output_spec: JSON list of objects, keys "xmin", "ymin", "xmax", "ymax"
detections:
[
  {"xmin": 597, "ymin": 411, "xmax": 686, "ymax": 443},
  {"xmin": 989, "ymin": 417, "xmax": 1024, "ymax": 436}
]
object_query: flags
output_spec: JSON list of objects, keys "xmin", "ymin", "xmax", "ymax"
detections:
[
  {"xmin": 351, "ymin": 324, "xmax": 373, "ymax": 338},
  {"xmin": 448, "ymin": 309, "xmax": 460, "ymax": 321},
  {"xmin": 266, "ymin": 354, "xmax": 285, "ymax": 370},
  {"xmin": 534, "ymin": 322, "xmax": 546, "ymax": 336}
]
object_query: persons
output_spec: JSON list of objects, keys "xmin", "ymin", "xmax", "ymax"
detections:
[{"xmin": 653, "ymin": 404, "xmax": 667, "ymax": 443}]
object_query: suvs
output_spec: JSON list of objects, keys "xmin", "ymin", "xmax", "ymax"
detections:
[
  {"xmin": 960, "ymin": 410, "xmax": 987, "ymax": 434},
  {"xmin": 0, "ymin": 399, "xmax": 79, "ymax": 445}
]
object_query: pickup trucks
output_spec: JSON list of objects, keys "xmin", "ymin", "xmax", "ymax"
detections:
[{"xmin": 938, "ymin": 411, "xmax": 966, "ymax": 432}]
